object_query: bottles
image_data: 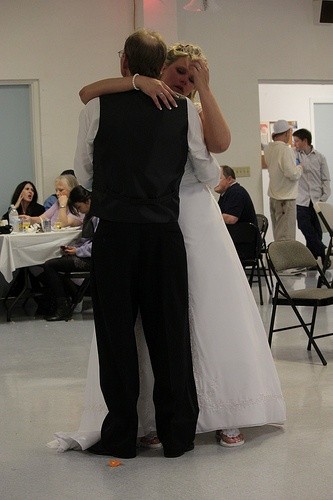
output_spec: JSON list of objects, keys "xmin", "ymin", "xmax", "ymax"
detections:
[
  {"xmin": 9, "ymin": 206, "xmax": 19, "ymax": 235},
  {"xmin": 8, "ymin": 204, "xmax": 14, "ymax": 214}
]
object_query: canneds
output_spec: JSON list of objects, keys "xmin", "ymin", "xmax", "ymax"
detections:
[{"xmin": 43, "ymin": 217, "xmax": 51, "ymax": 232}]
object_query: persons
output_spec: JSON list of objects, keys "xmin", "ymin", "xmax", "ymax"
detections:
[
  {"xmin": 1, "ymin": 181, "xmax": 45, "ymax": 300},
  {"xmin": 44, "ymin": 184, "xmax": 99, "ymax": 322},
  {"xmin": 43, "ymin": 169, "xmax": 76, "ymax": 212},
  {"xmin": 264, "ymin": 119, "xmax": 304, "ymax": 276},
  {"xmin": 47, "ymin": 27, "xmax": 288, "ymax": 454},
  {"xmin": 262, "ymin": 128, "xmax": 330, "ymax": 260},
  {"xmin": 18, "ymin": 174, "xmax": 79, "ymax": 322},
  {"xmin": 70, "ymin": 28, "xmax": 222, "ymax": 459},
  {"xmin": 215, "ymin": 166, "xmax": 261, "ymax": 265}
]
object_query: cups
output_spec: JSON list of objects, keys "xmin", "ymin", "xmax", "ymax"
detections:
[
  {"xmin": 18, "ymin": 217, "xmax": 24, "ymax": 234},
  {"xmin": 42, "ymin": 219, "xmax": 51, "ymax": 233},
  {"xmin": 54, "ymin": 222, "xmax": 62, "ymax": 232}
]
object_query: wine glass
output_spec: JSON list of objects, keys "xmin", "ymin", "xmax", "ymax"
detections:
[{"xmin": 23, "ymin": 217, "xmax": 31, "ymax": 234}]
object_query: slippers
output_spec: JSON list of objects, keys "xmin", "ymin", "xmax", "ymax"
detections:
[
  {"xmin": 216, "ymin": 428, "xmax": 244, "ymax": 447},
  {"xmin": 141, "ymin": 430, "xmax": 163, "ymax": 449}
]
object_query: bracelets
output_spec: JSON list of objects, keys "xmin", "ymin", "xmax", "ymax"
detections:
[
  {"xmin": 132, "ymin": 73, "xmax": 139, "ymax": 91},
  {"xmin": 59, "ymin": 205, "xmax": 66, "ymax": 208}
]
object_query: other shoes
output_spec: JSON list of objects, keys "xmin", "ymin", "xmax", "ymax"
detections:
[{"xmin": 278, "ymin": 267, "xmax": 301, "ymax": 276}]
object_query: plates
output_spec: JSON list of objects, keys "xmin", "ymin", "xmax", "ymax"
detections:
[{"xmin": 61, "ymin": 226, "xmax": 80, "ymax": 231}]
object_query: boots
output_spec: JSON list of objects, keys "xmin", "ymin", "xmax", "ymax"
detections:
[{"xmin": 46, "ymin": 297, "xmax": 70, "ymax": 320}]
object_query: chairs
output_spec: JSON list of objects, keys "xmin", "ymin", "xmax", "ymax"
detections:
[
  {"xmin": 314, "ymin": 202, "xmax": 333, "ymax": 289},
  {"xmin": 265, "ymin": 240, "xmax": 333, "ymax": 366},
  {"xmin": 241, "ymin": 214, "xmax": 274, "ymax": 304},
  {"xmin": 59, "ymin": 272, "xmax": 91, "ymax": 322}
]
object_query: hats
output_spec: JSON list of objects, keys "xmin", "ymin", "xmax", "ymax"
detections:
[{"xmin": 271, "ymin": 120, "xmax": 290, "ymax": 138}]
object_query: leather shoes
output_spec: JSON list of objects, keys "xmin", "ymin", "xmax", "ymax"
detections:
[
  {"xmin": 307, "ymin": 267, "xmax": 317, "ymax": 271},
  {"xmin": 86, "ymin": 442, "xmax": 107, "ymax": 455},
  {"xmin": 186, "ymin": 443, "xmax": 194, "ymax": 452},
  {"xmin": 321, "ymin": 251, "xmax": 331, "ymax": 269}
]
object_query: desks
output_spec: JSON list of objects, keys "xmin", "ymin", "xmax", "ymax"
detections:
[{"xmin": 0, "ymin": 225, "xmax": 83, "ymax": 321}]
